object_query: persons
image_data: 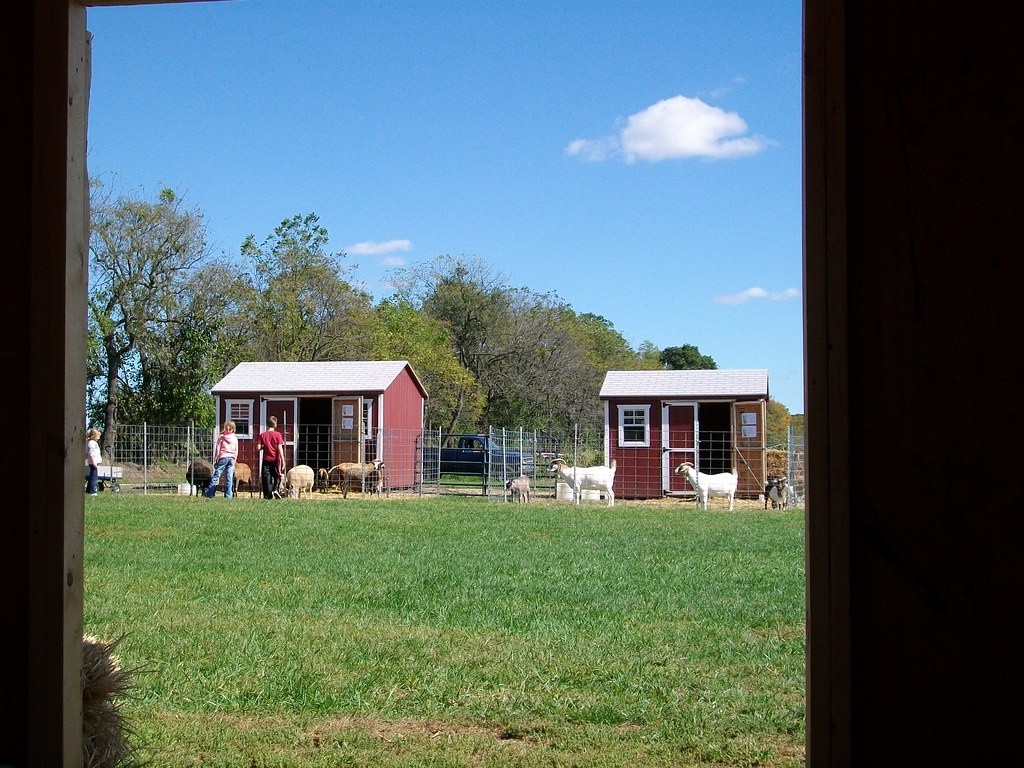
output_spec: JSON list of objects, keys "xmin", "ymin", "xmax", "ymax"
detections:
[
  {"xmin": 254, "ymin": 416, "xmax": 285, "ymax": 499},
  {"xmin": 86, "ymin": 429, "xmax": 102, "ymax": 496},
  {"xmin": 204, "ymin": 421, "xmax": 239, "ymax": 499}
]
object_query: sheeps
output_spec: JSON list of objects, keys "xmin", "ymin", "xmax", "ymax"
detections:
[
  {"xmin": 185, "ymin": 459, "xmax": 386, "ymax": 500},
  {"xmin": 503, "ymin": 475, "xmax": 530, "ymax": 503},
  {"xmin": 674, "ymin": 462, "xmax": 739, "ymax": 511},
  {"xmin": 546, "ymin": 458, "xmax": 617, "ymax": 508},
  {"xmin": 763, "ymin": 475, "xmax": 796, "ymax": 510}
]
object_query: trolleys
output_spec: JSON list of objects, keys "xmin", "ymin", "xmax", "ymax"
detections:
[{"xmin": 85, "ymin": 466, "xmax": 123, "ymax": 494}]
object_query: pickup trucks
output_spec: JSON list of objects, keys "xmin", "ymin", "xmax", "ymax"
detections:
[{"xmin": 423, "ymin": 434, "xmax": 535, "ymax": 481}]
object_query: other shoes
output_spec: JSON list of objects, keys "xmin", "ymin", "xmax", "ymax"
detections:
[
  {"xmin": 274, "ymin": 492, "xmax": 282, "ymax": 499},
  {"xmin": 89, "ymin": 493, "xmax": 97, "ymax": 496}
]
